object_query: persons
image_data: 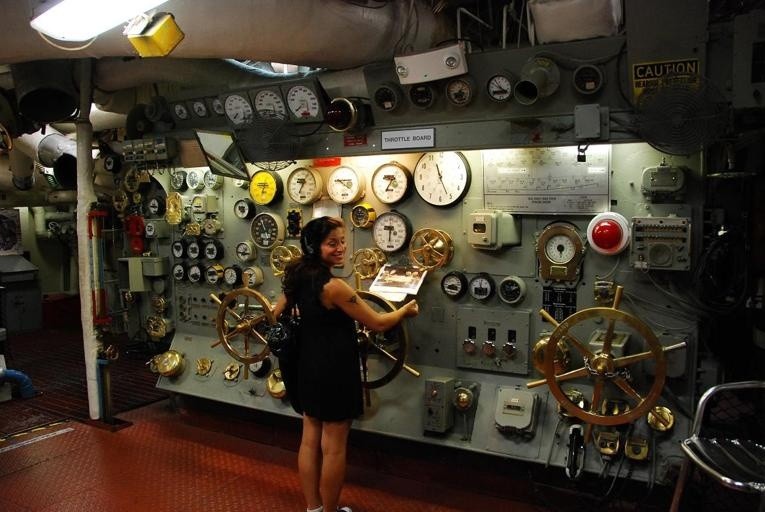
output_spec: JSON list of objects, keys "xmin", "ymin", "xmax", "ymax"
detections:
[{"xmin": 271, "ymin": 214, "xmax": 419, "ymax": 512}]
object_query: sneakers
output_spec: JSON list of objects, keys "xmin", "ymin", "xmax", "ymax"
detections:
[{"xmin": 307, "ymin": 504, "xmax": 353, "ymax": 512}]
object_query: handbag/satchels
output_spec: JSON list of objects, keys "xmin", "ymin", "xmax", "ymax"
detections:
[{"xmin": 269, "ymin": 315, "xmax": 303, "ymax": 357}]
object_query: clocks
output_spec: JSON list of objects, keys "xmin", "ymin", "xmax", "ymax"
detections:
[{"xmin": 411, "ymin": 150, "xmax": 472, "ymax": 209}]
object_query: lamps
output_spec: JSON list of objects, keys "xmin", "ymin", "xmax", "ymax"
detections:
[{"xmin": 28, "ymin": 0, "xmax": 168, "ymax": 42}]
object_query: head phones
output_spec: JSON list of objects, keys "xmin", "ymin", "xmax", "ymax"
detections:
[{"xmin": 301, "ymin": 235, "xmax": 314, "ymax": 256}]
